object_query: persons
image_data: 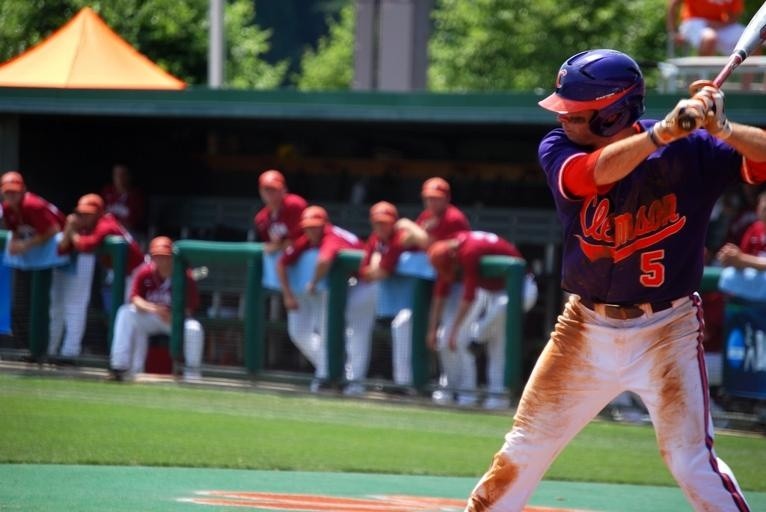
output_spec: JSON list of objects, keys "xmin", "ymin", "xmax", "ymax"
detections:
[
  {"xmin": 58, "ymin": 167, "xmax": 146, "ymax": 366},
  {"xmin": 253, "ymin": 170, "xmax": 329, "ymax": 394},
  {"xmin": 465, "ymin": 50, "xmax": 766, "ymax": 512},
  {"xmin": 666, "ymin": 0, "xmax": 764, "ymax": 56},
  {"xmin": 607, "ymin": 185, "xmax": 765, "ymax": 426},
  {"xmin": 2, "ymin": 171, "xmax": 96, "ymax": 367},
  {"xmin": 361, "ymin": 176, "xmax": 539, "ymax": 409},
  {"xmin": 279, "ymin": 206, "xmax": 376, "ymax": 393},
  {"xmin": 105, "ymin": 236, "xmax": 205, "ymax": 381}
]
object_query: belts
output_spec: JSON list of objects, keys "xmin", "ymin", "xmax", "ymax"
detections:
[{"xmin": 577, "ymin": 293, "xmax": 674, "ymax": 321}]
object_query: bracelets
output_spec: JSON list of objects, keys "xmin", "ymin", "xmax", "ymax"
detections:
[
  {"xmin": 648, "ymin": 124, "xmax": 667, "ymax": 148},
  {"xmin": 715, "ymin": 118, "xmax": 732, "ymax": 141}
]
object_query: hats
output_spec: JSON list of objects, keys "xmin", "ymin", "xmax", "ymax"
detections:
[
  {"xmin": 0, "ymin": 170, "xmax": 25, "ymax": 195},
  {"xmin": 419, "ymin": 175, "xmax": 451, "ymax": 201},
  {"xmin": 368, "ymin": 199, "xmax": 400, "ymax": 225},
  {"xmin": 299, "ymin": 203, "xmax": 330, "ymax": 231},
  {"xmin": 148, "ymin": 235, "xmax": 174, "ymax": 258},
  {"xmin": 73, "ymin": 191, "xmax": 105, "ymax": 216},
  {"xmin": 256, "ymin": 167, "xmax": 287, "ymax": 193}
]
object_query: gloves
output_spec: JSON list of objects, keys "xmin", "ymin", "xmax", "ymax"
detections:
[
  {"xmin": 688, "ymin": 77, "xmax": 735, "ymax": 145},
  {"xmin": 655, "ymin": 100, "xmax": 707, "ymax": 148}
]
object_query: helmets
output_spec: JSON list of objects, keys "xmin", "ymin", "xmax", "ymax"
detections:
[{"xmin": 538, "ymin": 46, "xmax": 645, "ymax": 138}]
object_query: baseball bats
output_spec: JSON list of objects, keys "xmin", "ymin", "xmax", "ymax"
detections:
[{"xmin": 677, "ymin": 4, "xmax": 766, "ymax": 132}]
